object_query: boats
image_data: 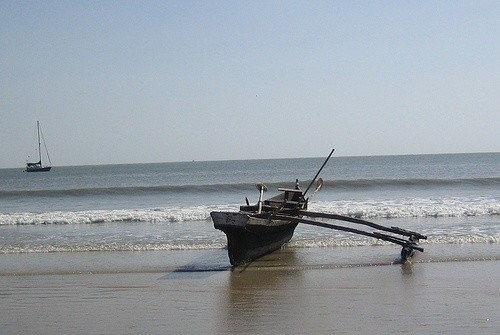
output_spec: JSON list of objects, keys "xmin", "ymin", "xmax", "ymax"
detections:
[
  {"xmin": 25, "ymin": 119, "xmax": 51, "ymax": 172},
  {"xmin": 212, "ymin": 147, "xmax": 309, "ymax": 265}
]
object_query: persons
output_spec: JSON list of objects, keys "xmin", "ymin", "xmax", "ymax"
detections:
[{"xmin": 296, "ymin": 179, "xmax": 299, "ymax": 190}]
